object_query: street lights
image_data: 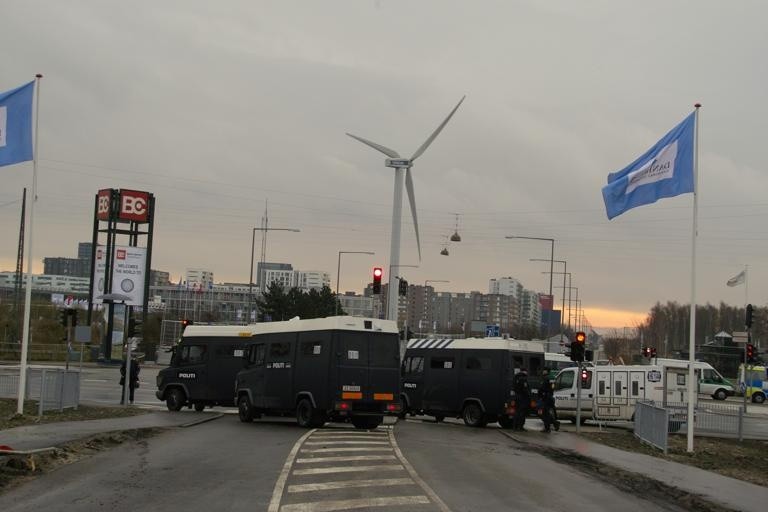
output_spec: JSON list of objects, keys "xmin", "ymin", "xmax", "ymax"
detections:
[
  {"xmin": 246, "ymin": 225, "xmax": 300, "ymax": 324},
  {"xmin": 387, "ymin": 264, "xmax": 419, "ymax": 322},
  {"xmin": 335, "ymin": 251, "xmax": 376, "ymax": 316},
  {"xmin": 420, "ymin": 278, "xmax": 449, "ymax": 339},
  {"xmin": 503, "ymin": 236, "xmax": 593, "ymax": 337}
]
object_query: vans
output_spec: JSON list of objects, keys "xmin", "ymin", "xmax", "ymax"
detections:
[{"xmin": 740, "ymin": 364, "xmax": 768, "ymax": 405}]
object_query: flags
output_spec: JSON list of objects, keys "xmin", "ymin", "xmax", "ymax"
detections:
[
  {"xmin": 727, "ymin": 270, "xmax": 744, "ymax": 287},
  {"xmin": 0, "ymin": 79, "xmax": 36, "ymax": 169},
  {"xmin": 601, "ymin": 109, "xmax": 697, "ymax": 221}
]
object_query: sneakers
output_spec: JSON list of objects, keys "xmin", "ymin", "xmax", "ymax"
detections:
[
  {"xmin": 541, "ymin": 421, "xmax": 560, "ymax": 432},
  {"xmin": 513, "ymin": 426, "xmax": 528, "ymax": 432}
]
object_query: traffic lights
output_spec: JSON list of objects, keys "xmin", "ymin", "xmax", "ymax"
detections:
[
  {"xmin": 746, "ymin": 304, "xmax": 755, "ymax": 327},
  {"xmin": 130, "ymin": 314, "xmax": 142, "ymax": 338},
  {"xmin": 745, "ymin": 341, "xmax": 754, "ymax": 359},
  {"xmin": 59, "ymin": 308, "xmax": 80, "ymax": 328},
  {"xmin": 371, "ymin": 264, "xmax": 384, "ymax": 296}
]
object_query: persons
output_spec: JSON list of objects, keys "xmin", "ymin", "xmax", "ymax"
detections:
[
  {"xmin": 120, "ymin": 355, "xmax": 140, "ymax": 405},
  {"xmin": 538, "ymin": 366, "xmax": 561, "ymax": 432},
  {"xmin": 513, "ymin": 368, "xmax": 533, "ymax": 432}
]
